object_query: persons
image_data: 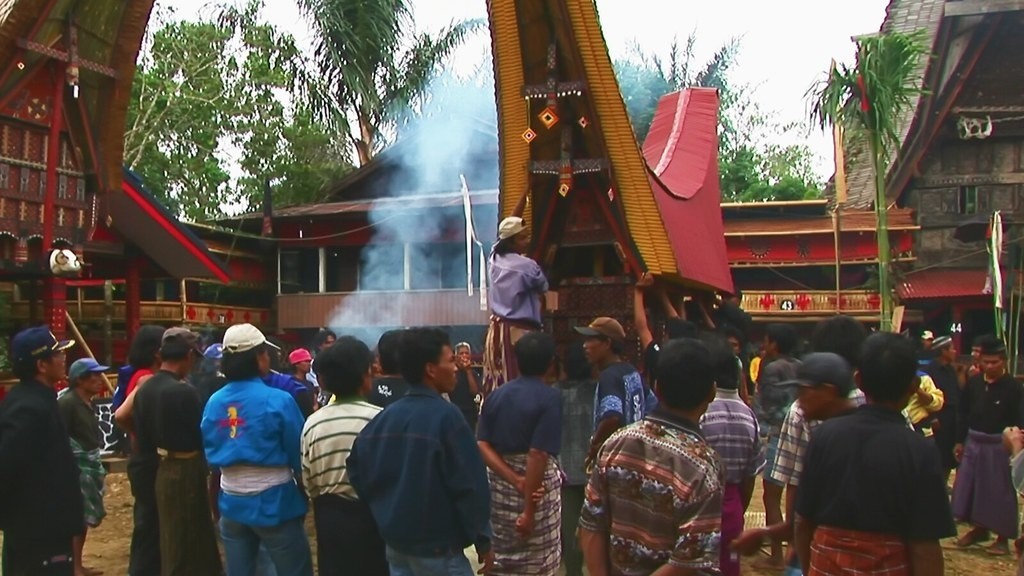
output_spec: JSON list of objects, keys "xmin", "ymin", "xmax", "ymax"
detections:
[{"xmin": 0, "ymin": 218, "xmax": 1024, "ymax": 576}]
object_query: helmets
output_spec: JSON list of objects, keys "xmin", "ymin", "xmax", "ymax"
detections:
[{"xmin": 288, "ymin": 348, "xmax": 312, "ymax": 367}]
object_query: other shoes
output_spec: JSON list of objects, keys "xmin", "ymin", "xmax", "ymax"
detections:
[{"xmin": 83, "ymin": 567, "xmax": 104, "ymax": 576}]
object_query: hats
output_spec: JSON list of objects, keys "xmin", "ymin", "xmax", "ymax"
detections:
[
  {"xmin": 203, "ymin": 342, "xmax": 223, "ymax": 358},
  {"xmin": 930, "ymin": 336, "xmax": 952, "ymax": 352},
  {"xmin": 161, "ymin": 327, "xmax": 206, "ymax": 360},
  {"xmin": 8, "ymin": 326, "xmax": 75, "ymax": 365},
  {"xmin": 573, "ymin": 317, "xmax": 628, "ymax": 345},
  {"xmin": 69, "ymin": 358, "xmax": 112, "ymax": 383},
  {"xmin": 774, "ymin": 352, "xmax": 850, "ymax": 400},
  {"xmin": 922, "ymin": 330, "xmax": 934, "ymax": 340},
  {"xmin": 222, "ymin": 323, "xmax": 282, "ymax": 355},
  {"xmin": 499, "ymin": 216, "xmax": 528, "ymax": 239}
]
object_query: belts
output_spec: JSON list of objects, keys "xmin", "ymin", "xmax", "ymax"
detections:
[{"xmin": 156, "ymin": 447, "xmax": 202, "ymax": 459}]
